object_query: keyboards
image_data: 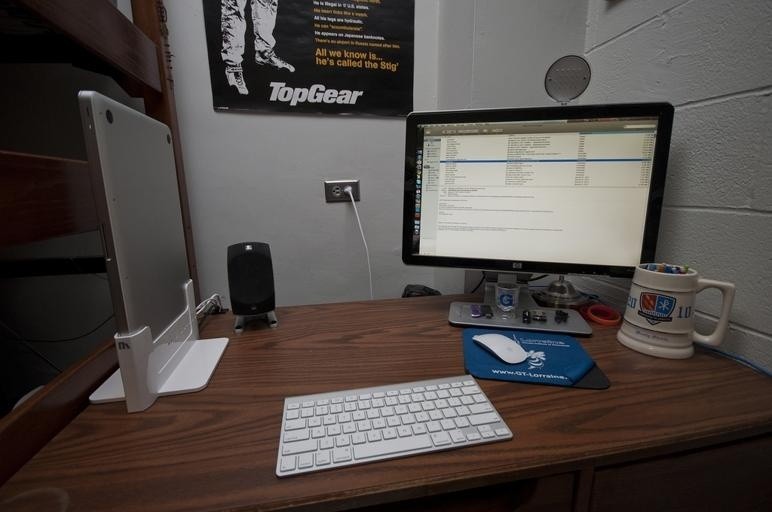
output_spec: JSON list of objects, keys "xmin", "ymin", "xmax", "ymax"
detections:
[{"xmin": 275, "ymin": 374, "xmax": 513, "ymax": 479}]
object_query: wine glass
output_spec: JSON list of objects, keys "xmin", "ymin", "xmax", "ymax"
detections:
[{"xmin": 494, "ymin": 281, "xmax": 520, "ymax": 320}]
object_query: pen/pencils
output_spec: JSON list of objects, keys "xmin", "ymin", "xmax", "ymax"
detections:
[{"xmin": 642, "ymin": 263, "xmax": 690, "ymax": 274}]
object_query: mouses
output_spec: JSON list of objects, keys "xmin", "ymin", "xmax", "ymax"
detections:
[{"xmin": 473, "ymin": 334, "xmax": 528, "ymax": 365}]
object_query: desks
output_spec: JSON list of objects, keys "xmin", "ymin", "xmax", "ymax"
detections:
[{"xmin": 0, "ymin": 289, "xmax": 772, "ymax": 512}]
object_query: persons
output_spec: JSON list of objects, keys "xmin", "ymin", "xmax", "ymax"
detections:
[{"xmin": 220, "ymin": 0, "xmax": 296, "ymax": 97}]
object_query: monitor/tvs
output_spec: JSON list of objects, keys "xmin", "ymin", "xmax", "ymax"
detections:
[
  {"xmin": 402, "ymin": 102, "xmax": 674, "ymax": 337},
  {"xmin": 77, "ymin": 91, "xmax": 201, "ymax": 413}
]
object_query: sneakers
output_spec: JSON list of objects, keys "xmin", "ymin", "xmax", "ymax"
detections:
[
  {"xmin": 224, "ymin": 66, "xmax": 251, "ymax": 98},
  {"xmin": 253, "ymin": 50, "xmax": 296, "ymax": 74}
]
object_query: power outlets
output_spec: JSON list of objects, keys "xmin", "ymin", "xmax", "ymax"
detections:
[{"xmin": 324, "ymin": 180, "xmax": 360, "ymax": 203}]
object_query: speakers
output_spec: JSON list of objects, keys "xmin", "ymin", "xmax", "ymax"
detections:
[{"xmin": 226, "ymin": 242, "xmax": 275, "ymax": 315}]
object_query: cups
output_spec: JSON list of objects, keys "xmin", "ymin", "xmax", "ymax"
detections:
[{"xmin": 617, "ymin": 263, "xmax": 735, "ymax": 360}]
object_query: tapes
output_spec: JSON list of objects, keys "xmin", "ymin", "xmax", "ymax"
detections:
[{"xmin": 587, "ymin": 304, "xmax": 621, "ymax": 326}]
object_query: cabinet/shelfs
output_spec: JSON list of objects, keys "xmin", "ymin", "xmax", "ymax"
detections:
[{"xmin": 576, "ymin": 436, "xmax": 772, "ymax": 512}]
